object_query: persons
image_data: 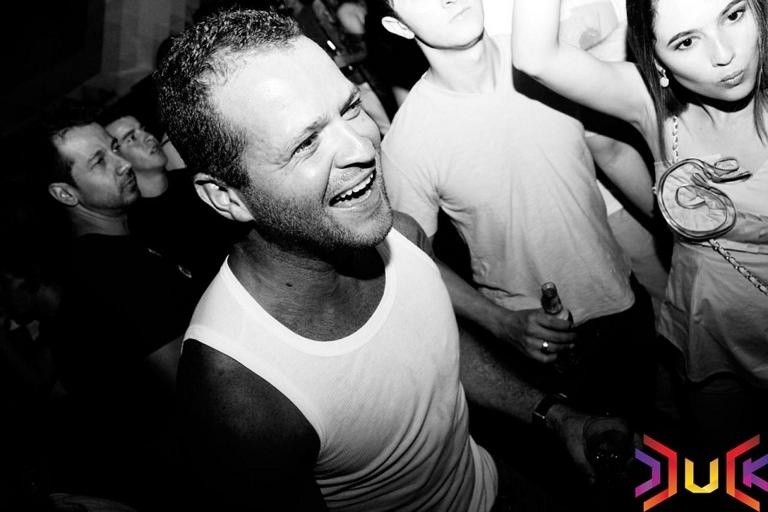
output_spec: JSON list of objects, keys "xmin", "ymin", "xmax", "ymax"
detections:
[{"xmin": 39, "ymin": 0, "xmax": 768, "ymax": 512}]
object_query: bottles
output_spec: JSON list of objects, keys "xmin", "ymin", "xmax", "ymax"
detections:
[{"xmin": 540, "ymin": 282, "xmax": 575, "ymax": 332}]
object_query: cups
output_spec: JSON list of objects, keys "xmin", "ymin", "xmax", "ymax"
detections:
[{"xmin": 582, "ymin": 412, "xmax": 637, "ymax": 482}]
